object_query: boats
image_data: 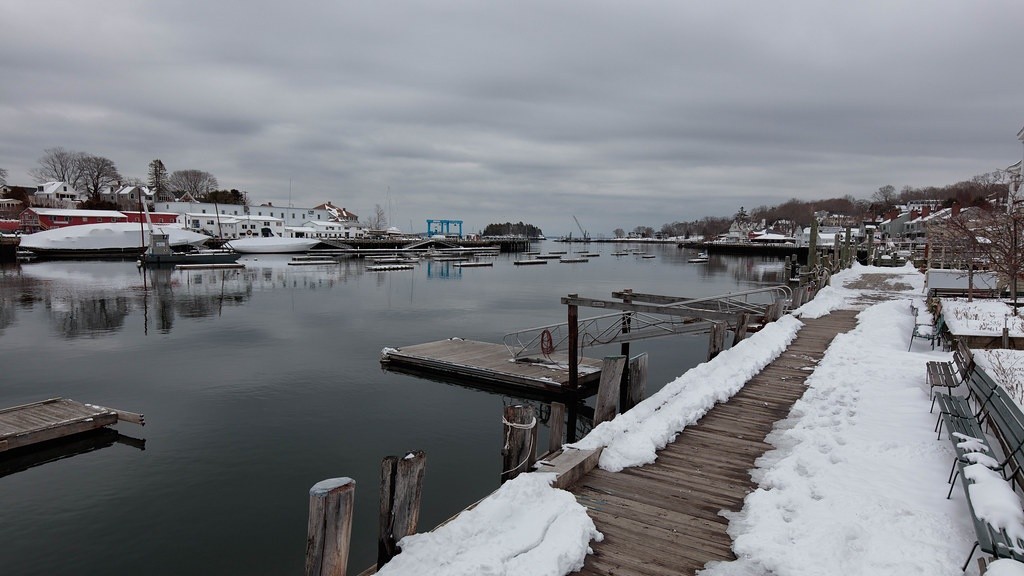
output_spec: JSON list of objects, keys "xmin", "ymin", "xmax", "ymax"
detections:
[
  {"xmin": 184, "ymin": 227, "xmax": 230, "ymax": 250},
  {"xmin": 688, "ymin": 252, "xmax": 708, "ymax": 263},
  {"xmin": 220, "ymin": 226, "xmax": 323, "ymax": 253},
  {"xmin": 140, "ymin": 194, "xmax": 241, "ymax": 265}
]
